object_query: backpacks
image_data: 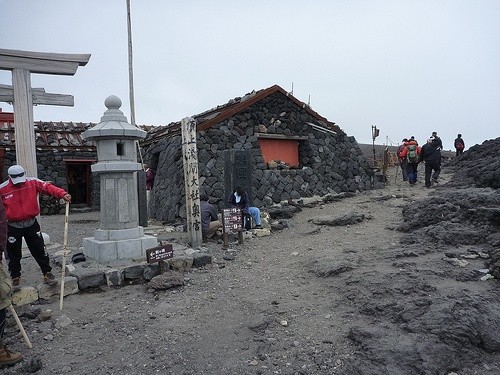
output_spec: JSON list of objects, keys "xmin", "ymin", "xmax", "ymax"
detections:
[
  {"xmin": 407, "ymin": 145, "xmax": 417, "ymax": 162},
  {"xmin": 456, "ymin": 142, "xmax": 463, "ymax": 149}
]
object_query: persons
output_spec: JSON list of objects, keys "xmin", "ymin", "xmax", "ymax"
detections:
[
  {"xmin": 143, "ymin": 164, "xmax": 154, "ymax": 208},
  {"xmin": 200, "ymin": 194, "xmax": 221, "ymax": 241},
  {"xmin": 420, "ymin": 136, "xmax": 441, "ymax": 189},
  {"xmin": 0, "ymin": 165, "xmax": 71, "ymax": 291},
  {"xmin": 226, "ymin": 186, "xmax": 263, "ymax": 229},
  {"xmin": 0, "ymin": 196, "xmax": 23, "ymax": 364},
  {"xmin": 454, "ymin": 134, "xmax": 465, "ymax": 156},
  {"xmin": 432, "ymin": 132, "xmax": 443, "ymax": 166},
  {"xmin": 396, "ymin": 136, "xmax": 421, "ymax": 186}
]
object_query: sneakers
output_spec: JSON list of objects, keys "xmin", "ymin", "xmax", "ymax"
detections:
[
  {"xmin": 12, "ymin": 277, "xmax": 21, "ymax": 291},
  {"xmin": 44, "ymin": 272, "xmax": 58, "ymax": 285}
]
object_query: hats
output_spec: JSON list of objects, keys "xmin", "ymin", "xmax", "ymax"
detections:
[{"xmin": 8, "ymin": 165, "xmax": 26, "ymax": 184}]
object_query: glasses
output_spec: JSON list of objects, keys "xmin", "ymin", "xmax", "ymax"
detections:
[{"xmin": 8, "ymin": 172, "xmax": 24, "ymax": 178}]
